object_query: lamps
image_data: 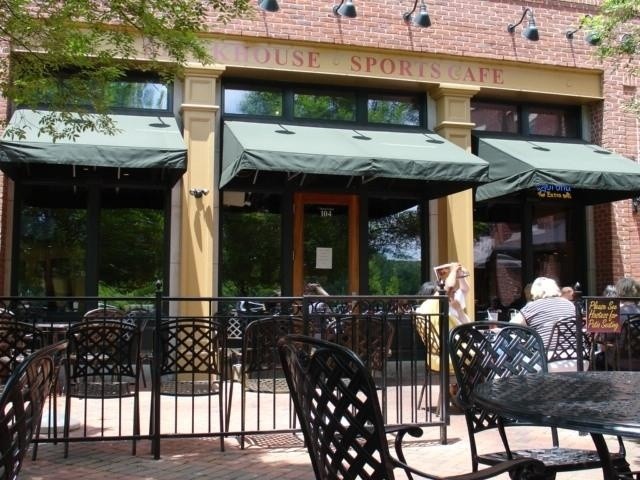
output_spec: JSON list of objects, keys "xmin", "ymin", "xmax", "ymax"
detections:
[
  {"xmin": 331, "ymin": 0, "xmax": 358, "ymax": 17},
  {"xmin": 255, "ymin": 0, "xmax": 281, "ymax": 13},
  {"xmin": 189, "ymin": 187, "xmax": 209, "ymax": 199},
  {"xmin": 507, "ymin": 6, "xmax": 540, "ymax": 41},
  {"xmin": 403, "ymin": 0, "xmax": 432, "ymax": 27},
  {"xmin": 565, "ymin": 14, "xmax": 601, "ymax": 47}
]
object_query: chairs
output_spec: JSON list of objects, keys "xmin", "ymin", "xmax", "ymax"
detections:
[
  {"xmin": 2, "ymin": 340, "xmax": 70, "ymax": 476},
  {"xmin": 277, "ymin": 335, "xmax": 557, "ymax": 480},
  {"xmin": 64, "ymin": 303, "xmax": 444, "ymax": 461},
  {"xmin": 0, "ymin": 318, "xmax": 44, "ymax": 389},
  {"xmin": 450, "ymin": 321, "xmax": 633, "ymax": 480}
]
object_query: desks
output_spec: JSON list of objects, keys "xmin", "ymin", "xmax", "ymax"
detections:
[{"xmin": 472, "ymin": 370, "xmax": 640, "ymax": 475}]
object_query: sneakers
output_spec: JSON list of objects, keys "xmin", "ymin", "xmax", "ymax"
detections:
[{"xmin": 449, "ymin": 384, "xmax": 458, "ymax": 405}]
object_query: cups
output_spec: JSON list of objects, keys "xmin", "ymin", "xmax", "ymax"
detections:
[
  {"xmin": 510, "ymin": 312, "xmax": 516, "ymax": 319},
  {"xmin": 487, "ymin": 310, "xmax": 499, "ymax": 330},
  {"xmin": 73, "ymin": 302, "xmax": 79, "ymax": 312}
]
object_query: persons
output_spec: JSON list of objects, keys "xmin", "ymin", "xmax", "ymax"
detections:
[
  {"xmin": 414, "ymin": 262, "xmax": 640, "ymax": 410},
  {"xmin": 304, "ymin": 284, "xmax": 395, "ymax": 357}
]
